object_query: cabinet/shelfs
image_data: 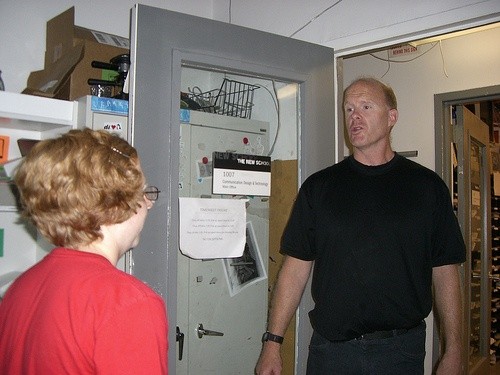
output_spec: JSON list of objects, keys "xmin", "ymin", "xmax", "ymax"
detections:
[{"xmin": 80, "ymin": 94, "xmax": 270, "ymax": 375}]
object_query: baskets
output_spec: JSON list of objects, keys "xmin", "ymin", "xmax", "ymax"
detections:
[{"xmin": 192, "ymin": 78, "xmax": 260, "ymax": 120}]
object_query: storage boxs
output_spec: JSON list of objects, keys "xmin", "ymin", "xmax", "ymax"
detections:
[{"xmin": 46, "ymin": 5, "xmax": 130, "ymax": 102}]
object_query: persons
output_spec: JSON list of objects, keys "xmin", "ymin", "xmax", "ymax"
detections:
[
  {"xmin": 254, "ymin": 77, "xmax": 467, "ymax": 375},
  {"xmin": 0, "ymin": 128, "xmax": 169, "ymax": 375}
]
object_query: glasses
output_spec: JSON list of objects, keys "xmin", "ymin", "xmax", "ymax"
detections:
[{"xmin": 143, "ymin": 186, "xmax": 161, "ymax": 201}]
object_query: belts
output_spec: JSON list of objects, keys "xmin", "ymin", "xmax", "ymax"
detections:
[{"xmin": 353, "ymin": 320, "xmax": 424, "ymax": 340}]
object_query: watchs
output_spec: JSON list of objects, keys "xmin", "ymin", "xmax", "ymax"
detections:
[{"xmin": 262, "ymin": 331, "xmax": 284, "ymax": 344}]
